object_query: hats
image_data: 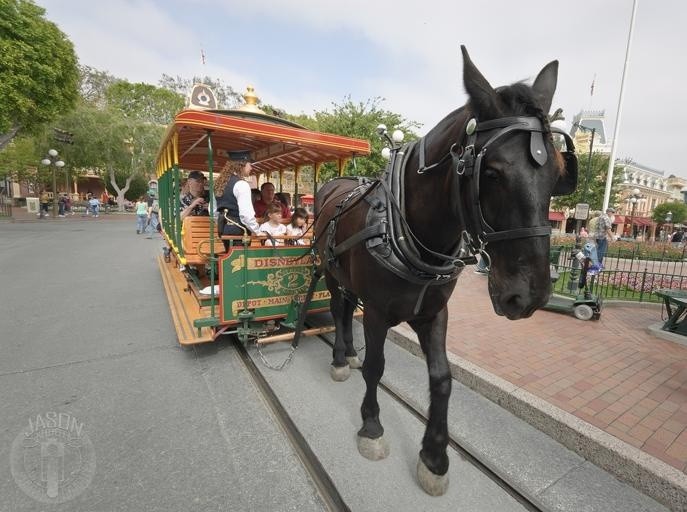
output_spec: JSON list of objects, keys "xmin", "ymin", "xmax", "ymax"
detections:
[
  {"xmin": 189, "ymin": 171, "xmax": 207, "ymax": 181},
  {"xmin": 227, "ymin": 150, "xmax": 257, "ymax": 162}
]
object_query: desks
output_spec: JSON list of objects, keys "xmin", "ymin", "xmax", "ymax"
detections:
[{"xmin": 656, "ymin": 289, "xmax": 687, "ymax": 336}]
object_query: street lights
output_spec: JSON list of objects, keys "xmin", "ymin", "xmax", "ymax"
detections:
[
  {"xmin": 41, "ymin": 149, "xmax": 65, "ymax": 217},
  {"xmin": 663, "ymin": 211, "xmax": 673, "ymax": 244},
  {"xmin": 625, "ymin": 188, "xmax": 647, "ymax": 235}
]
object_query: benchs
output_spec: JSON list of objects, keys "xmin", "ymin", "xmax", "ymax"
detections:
[{"xmin": 182, "ymin": 216, "xmax": 314, "ymax": 264}]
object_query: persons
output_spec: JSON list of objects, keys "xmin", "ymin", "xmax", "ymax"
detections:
[
  {"xmin": 207, "ymin": 149, "xmax": 266, "ymax": 253},
  {"xmin": 57, "ymin": 193, "xmax": 76, "ymax": 218},
  {"xmin": 87, "ymin": 196, "xmax": 101, "ymax": 218},
  {"xmin": 145, "ymin": 190, "xmax": 160, "ymax": 239},
  {"xmin": 35, "ymin": 191, "xmax": 49, "ymax": 217},
  {"xmin": 654, "ymin": 223, "xmax": 686, "ymax": 250},
  {"xmin": 595, "ymin": 207, "xmax": 615, "ymax": 265},
  {"xmin": 172, "ymin": 169, "xmax": 309, "ymax": 284},
  {"xmin": 107, "ymin": 196, "xmax": 136, "ymax": 212},
  {"xmin": 134, "ymin": 196, "xmax": 148, "ymax": 235},
  {"xmin": 588, "ymin": 210, "xmax": 600, "ymax": 232}
]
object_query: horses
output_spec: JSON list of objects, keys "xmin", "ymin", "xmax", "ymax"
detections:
[{"xmin": 312, "ymin": 44, "xmax": 567, "ymax": 497}]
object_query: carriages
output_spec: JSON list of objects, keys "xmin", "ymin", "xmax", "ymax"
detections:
[{"xmin": 148, "ymin": 44, "xmax": 579, "ymax": 498}]
